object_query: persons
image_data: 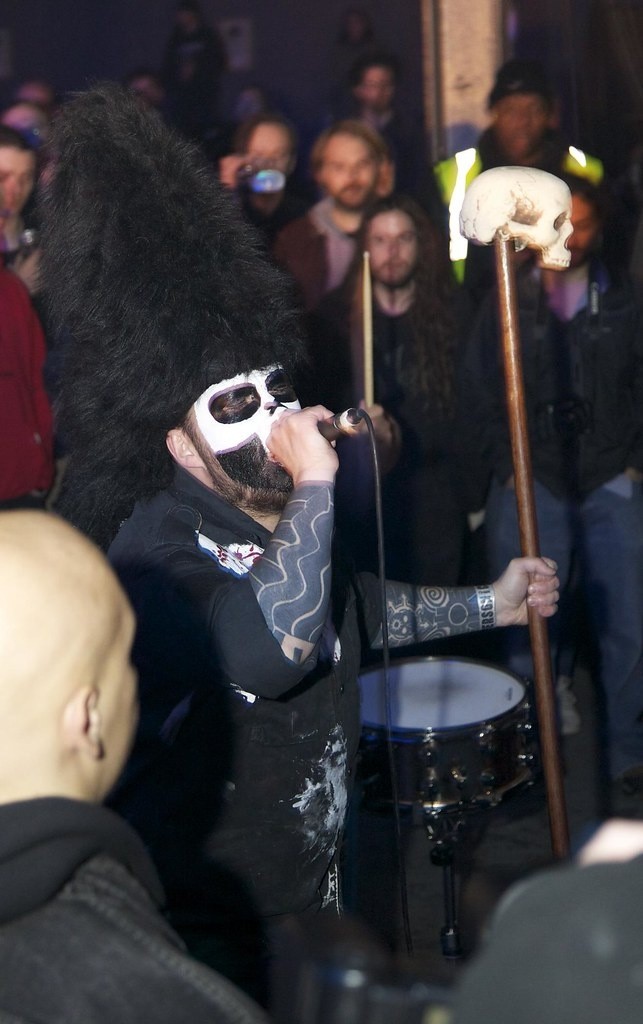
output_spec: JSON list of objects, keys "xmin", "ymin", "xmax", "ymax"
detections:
[
  {"xmin": 0, "ymin": 118, "xmax": 52, "ymax": 337},
  {"xmin": 122, "ymin": 65, "xmax": 169, "ymax": 115},
  {"xmin": 368, "ymin": 129, "xmax": 398, "ymax": 199},
  {"xmin": 335, "ymin": 6, "xmax": 381, "ymax": 116},
  {"xmin": 3, "ymin": 97, "xmax": 52, "ymax": 152},
  {"xmin": 451, "ymin": 165, "xmax": 643, "ymax": 850},
  {"xmin": 331, "ymin": 47, "xmax": 436, "ymax": 212},
  {"xmin": 270, "ymin": 117, "xmax": 390, "ymax": 319},
  {"xmin": 418, "ymin": 51, "xmax": 614, "ymax": 325},
  {"xmin": 12, "ymin": 78, "xmax": 56, "ymax": 108},
  {"xmin": 0, "ymin": 261, "xmax": 67, "ymax": 509},
  {"xmin": 299, "ymin": 190, "xmax": 498, "ymax": 666},
  {"xmin": 211, "ymin": 107, "xmax": 323, "ymax": 245},
  {"xmin": 159, "ymin": 0, "xmax": 226, "ymax": 162},
  {"xmin": 218, "ymin": 79, "xmax": 270, "ymax": 137},
  {"xmin": 96, "ymin": 334, "xmax": 567, "ymax": 1023},
  {"xmin": 0, "ymin": 506, "xmax": 282, "ymax": 1023}
]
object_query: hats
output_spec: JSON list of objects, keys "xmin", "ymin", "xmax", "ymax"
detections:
[{"xmin": 483, "ymin": 59, "xmax": 553, "ymax": 114}]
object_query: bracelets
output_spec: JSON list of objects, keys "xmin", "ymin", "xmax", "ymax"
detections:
[{"xmin": 472, "ymin": 580, "xmax": 500, "ymax": 634}]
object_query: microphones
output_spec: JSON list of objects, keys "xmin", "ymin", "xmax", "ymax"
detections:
[{"xmin": 316, "ymin": 405, "xmax": 368, "ymax": 442}]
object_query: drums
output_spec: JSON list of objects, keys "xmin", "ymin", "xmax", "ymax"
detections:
[{"xmin": 352, "ymin": 652, "xmax": 537, "ymax": 820}]
